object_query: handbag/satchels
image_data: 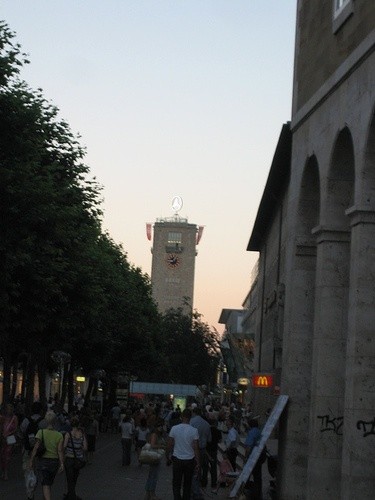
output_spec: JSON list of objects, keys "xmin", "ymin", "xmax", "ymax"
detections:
[
  {"xmin": 73, "ymin": 450, "xmax": 88, "ymax": 468},
  {"xmin": 25, "ymin": 469, "xmax": 38, "ymax": 500},
  {"xmin": 35, "ymin": 428, "xmax": 46, "ymax": 457},
  {"xmin": 139, "ymin": 444, "xmax": 163, "ymax": 464}
]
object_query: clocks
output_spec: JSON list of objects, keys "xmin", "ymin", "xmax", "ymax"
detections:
[{"xmin": 167, "ymin": 254, "xmax": 182, "ymax": 269}]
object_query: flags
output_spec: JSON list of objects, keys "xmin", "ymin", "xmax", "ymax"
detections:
[
  {"xmin": 197, "ymin": 227, "xmax": 204, "ymax": 244},
  {"xmin": 146, "ymin": 223, "xmax": 151, "ymax": 240}
]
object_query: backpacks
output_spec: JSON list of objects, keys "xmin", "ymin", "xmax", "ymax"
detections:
[{"xmin": 24, "ymin": 416, "xmax": 46, "ymax": 451}]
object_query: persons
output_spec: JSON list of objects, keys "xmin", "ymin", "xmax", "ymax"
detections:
[
  {"xmin": 0, "ymin": 392, "xmax": 99, "ymax": 500},
  {"xmin": 107, "ymin": 390, "xmax": 263, "ymax": 500}
]
